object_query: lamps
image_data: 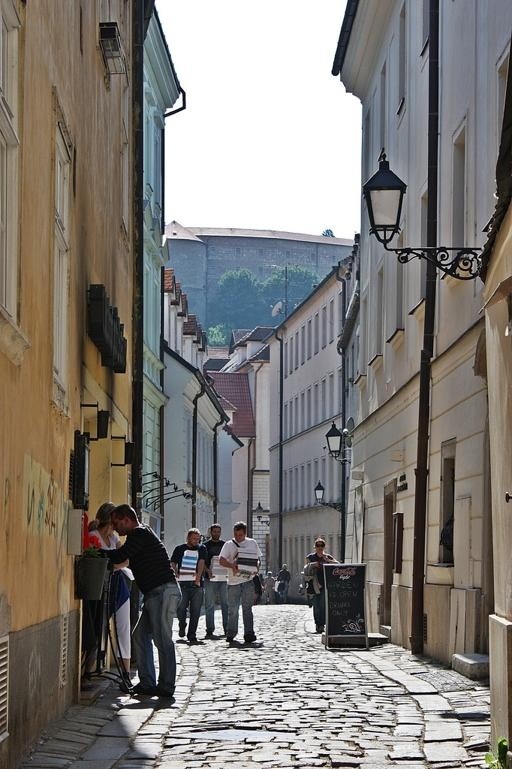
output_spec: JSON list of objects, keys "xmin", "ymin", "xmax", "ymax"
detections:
[
  {"xmin": 359, "ymin": 150, "xmax": 483, "ymax": 281},
  {"xmin": 324, "ymin": 419, "xmax": 347, "ymax": 464},
  {"xmin": 254, "ymin": 501, "xmax": 269, "ymax": 525},
  {"xmin": 314, "ymin": 479, "xmax": 341, "ymax": 513},
  {"xmin": 142, "ymin": 470, "xmax": 195, "ymax": 512}
]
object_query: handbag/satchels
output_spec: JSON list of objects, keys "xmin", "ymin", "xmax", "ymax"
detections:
[
  {"xmin": 253, "ymin": 575, "xmax": 261, "ymax": 593},
  {"xmin": 305, "ymin": 563, "xmax": 316, "ymax": 594},
  {"xmin": 111, "ymin": 571, "xmax": 131, "ymax": 614}
]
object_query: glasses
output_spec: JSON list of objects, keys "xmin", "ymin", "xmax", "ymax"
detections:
[{"xmin": 316, "ymin": 544, "xmax": 324, "ymax": 547}]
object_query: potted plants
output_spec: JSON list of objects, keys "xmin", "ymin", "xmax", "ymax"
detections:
[{"xmin": 74, "ymin": 545, "xmax": 111, "ymax": 602}]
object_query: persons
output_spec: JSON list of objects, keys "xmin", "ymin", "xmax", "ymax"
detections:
[
  {"xmin": 91, "ymin": 503, "xmax": 184, "ymax": 699},
  {"xmin": 253, "ymin": 562, "xmax": 291, "ymax": 606},
  {"xmin": 169, "ymin": 527, "xmax": 211, "ymax": 642},
  {"xmin": 87, "ymin": 501, "xmax": 136, "ymax": 694},
  {"xmin": 199, "ymin": 523, "xmax": 230, "ymax": 640},
  {"xmin": 216, "ymin": 521, "xmax": 264, "ymax": 643},
  {"xmin": 303, "ymin": 537, "xmax": 340, "ymax": 634}
]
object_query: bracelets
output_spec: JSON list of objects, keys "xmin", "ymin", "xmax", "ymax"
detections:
[{"xmin": 196, "ymin": 576, "xmax": 201, "ymax": 582}]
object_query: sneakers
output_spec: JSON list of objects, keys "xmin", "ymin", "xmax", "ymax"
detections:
[
  {"xmin": 179, "ymin": 627, "xmax": 185, "ymax": 637},
  {"xmin": 187, "ymin": 633, "xmax": 197, "ymax": 643},
  {"xmin": 226, "ymin": 636, "xmax": 233, "ymax": 642},
  {"xmin": 244, "ymin": 634, "xmax": 256, "ymax": 641},
  {"xmin": 131, "ymin": 684, "xmax": 154, "ymax": 694},
  {"xmin": 83, "ymin": 674, "xmax": 92, "ymax": 687}
]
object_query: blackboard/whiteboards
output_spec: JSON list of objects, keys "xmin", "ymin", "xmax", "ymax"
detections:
[{"xmin": 323, "ymin": 563, "xmax": 368, "ymax": 638}]
